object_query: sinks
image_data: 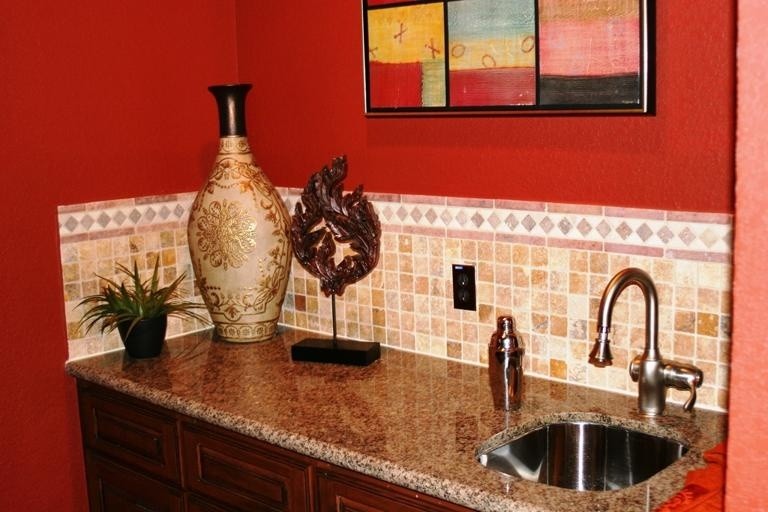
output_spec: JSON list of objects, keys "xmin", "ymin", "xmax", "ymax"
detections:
[{"xmin": 476, "ymin": 410, "xmax": 692, "ymax": 490}]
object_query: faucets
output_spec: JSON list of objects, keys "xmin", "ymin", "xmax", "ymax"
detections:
[{"xmin": 587, "ymin": 266, "xmax": 704, "ymax": 415}]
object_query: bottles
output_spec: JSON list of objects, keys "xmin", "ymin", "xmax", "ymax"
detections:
[{"xmin": 489, "ymin": 315, "xmax": 525, "ymax": 413}]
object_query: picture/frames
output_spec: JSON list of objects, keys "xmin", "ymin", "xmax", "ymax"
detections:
[{"xmin": 360, "ymin": 1, "xmax": 656, "ymax": 119}]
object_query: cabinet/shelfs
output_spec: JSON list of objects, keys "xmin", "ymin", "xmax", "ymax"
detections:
[
  {"xmin": 312, "ymin": 457, "xmax": 479, "ymax": 511},
  {"xmin": 177, "ymin": 415, "xmax": 313, "ymax": 510},
  {"xmin": 76, "ymin": 381, "xmax": 186, "ymax": 511}
]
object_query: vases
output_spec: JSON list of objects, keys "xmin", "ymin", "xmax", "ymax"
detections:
[{"xmin": 185, "ymin": 83, "xmax": 294, "ymax": 344}]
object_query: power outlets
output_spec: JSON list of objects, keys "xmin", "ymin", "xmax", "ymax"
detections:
[{"xmin": 451, "ymin": 264, "xmax": 478, "ymax": 313}]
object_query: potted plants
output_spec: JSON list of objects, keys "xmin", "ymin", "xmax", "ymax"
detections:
[{"xmin": 70, "ymin": 253, "xmax": 215, "ymax": 362}]
